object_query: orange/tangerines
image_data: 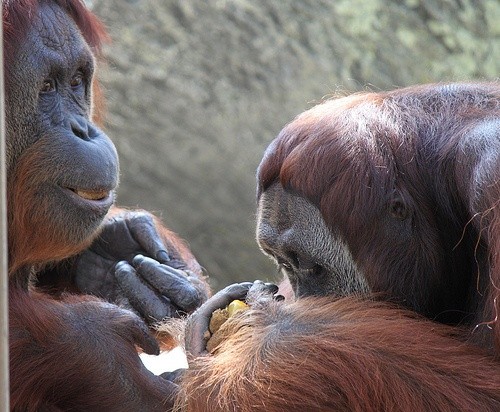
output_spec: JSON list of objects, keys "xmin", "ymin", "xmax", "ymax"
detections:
[{"xmin": 226, "ymin": 300, "xmax": 250, "ymax": 316}]
